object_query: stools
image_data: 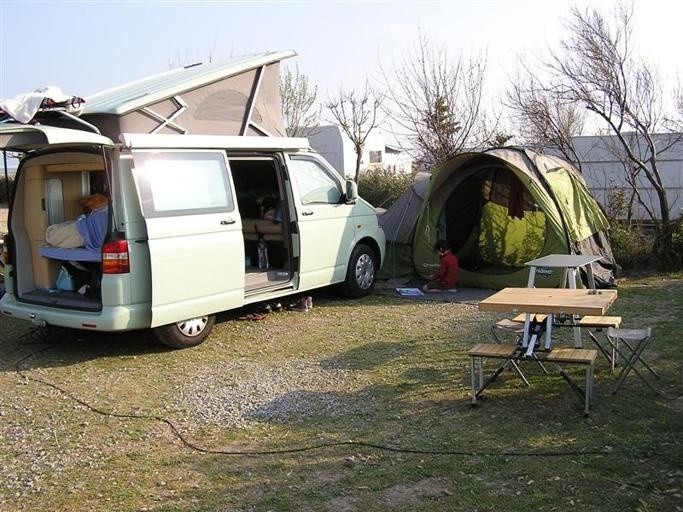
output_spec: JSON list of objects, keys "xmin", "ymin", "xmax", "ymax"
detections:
[
  {"xmin": 491, "ymin": 317, "xmax": 550, "ymax": 387},
  {"xmin": 607, "ymin": 328, "xmax": 660, "ymax": 396}
]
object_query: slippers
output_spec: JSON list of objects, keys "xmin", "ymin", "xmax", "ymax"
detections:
[{"xmin": 237, "ymin": 311, "xmax": 264, "ymax": 320}]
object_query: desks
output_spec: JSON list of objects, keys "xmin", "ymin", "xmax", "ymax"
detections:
[
  {"xmin": 478, "ymin": 287, "xmax": 619, "ymax": 352},
  {"xmin": 524, "ymin": 253, "xmax": 604, "ymax": 349}
]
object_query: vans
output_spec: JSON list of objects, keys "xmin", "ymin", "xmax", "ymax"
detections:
[{"xmin": 0, "ymin": 49, "xmax": 386, "ymax": 348}]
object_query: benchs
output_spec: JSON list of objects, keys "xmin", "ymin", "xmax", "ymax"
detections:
[
  {"xmin": 513, "ymin": 313, "xmax": 623, "ymax": 372},
  {"xmin": 467, "ymin": 344, "xmax": 598, "ymax": 419}
]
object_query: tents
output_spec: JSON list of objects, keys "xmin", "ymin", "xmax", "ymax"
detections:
[{"xmin": 374, "ymin": 140, "xmax": 622, "ymax": 295}]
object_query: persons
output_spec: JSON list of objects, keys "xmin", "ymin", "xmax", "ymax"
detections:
[{"xmin": 420, "ymin": 239, "xmax": 458, "ymax": 295}]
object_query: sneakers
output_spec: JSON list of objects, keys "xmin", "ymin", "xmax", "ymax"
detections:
[
  {"xmin": 288, "ymin": 296, "xmax": 314, "ymax": 313},
  {"xmin": 261, "ymin": 303, "xmax": 282, "ymax": 314}
]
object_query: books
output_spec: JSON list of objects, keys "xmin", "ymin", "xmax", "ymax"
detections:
[{"xmin": 396, "ymin": 286, "xmax": 424, "ymax": 297}]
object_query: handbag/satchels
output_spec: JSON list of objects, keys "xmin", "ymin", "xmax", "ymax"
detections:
[{"xmin": 56, "ymin": 266, "xmax": 81, "ymax": 291}]
